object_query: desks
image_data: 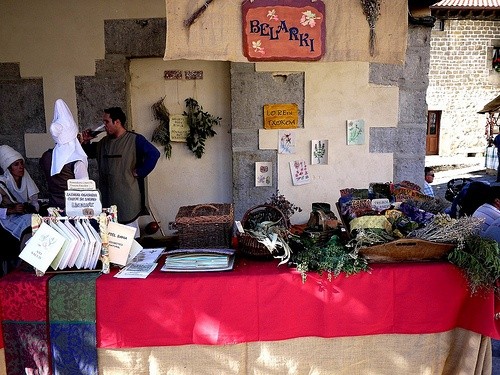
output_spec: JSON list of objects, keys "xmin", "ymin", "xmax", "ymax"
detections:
[{"xmin": 0, "ymin": 237, "xmax": 500, "ymax": 375}]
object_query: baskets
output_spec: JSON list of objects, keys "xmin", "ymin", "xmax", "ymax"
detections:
[
  {"xmin": 175, "ymin": 203, "xmax": 234, "ymax": 249},
  {"xmin": 334, "ymin": 194, "xmax": 458, "ymax": 263},
  {"xmin": 235, "ymin": 204, "xmax": 291, "ymax": 262},
  {"xmin": 302, "ymin": 209, "xmax": 342, "ymax": 248}
]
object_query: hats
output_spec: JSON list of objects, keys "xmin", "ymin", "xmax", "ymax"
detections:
[
  {"xmin": 50, "ymin": 99, "xmax": 89, "ymax": 176},
  {"xmin": 0, "ymin": 145, "xmax": 40, "ymax": 203}
]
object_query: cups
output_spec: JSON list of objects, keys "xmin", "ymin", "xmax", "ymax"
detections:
[{"xmin": 87, "ymin": 121, "xmax": 108, "ymax": 138}]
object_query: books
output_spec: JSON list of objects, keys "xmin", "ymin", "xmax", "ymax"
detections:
[{"xmin": 19, "ymin": 221, "xmax": 167, "ymax": 278}]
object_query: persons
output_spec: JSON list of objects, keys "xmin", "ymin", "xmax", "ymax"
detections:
[
  {"xmin": 0, "ymin": 153, "xmax": 39, "ymax": 269},
  {"xmin": 38, "ymin": 121, "xmax": 89, "ymax": 217},
  {"xmin": 423, "ymin": 167, "xmax": 434, "ymax": 198},
  {"xmin": 82, "ymin": 107, "xmax": 160, "ymax": 243}
]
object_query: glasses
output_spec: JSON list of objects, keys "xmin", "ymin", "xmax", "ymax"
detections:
[{"xmin": 427, "ymin": 174, "xmax": 434, "ymax": 177}]
